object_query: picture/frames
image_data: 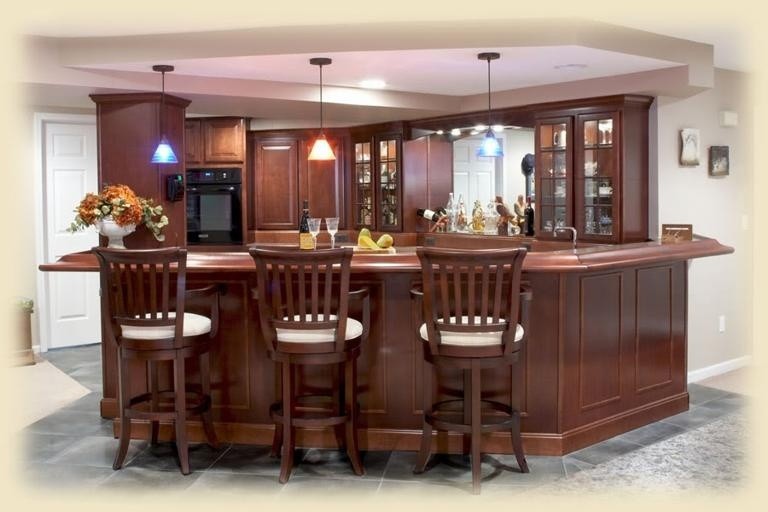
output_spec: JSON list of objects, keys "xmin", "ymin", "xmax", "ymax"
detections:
[
  {"xmin": 680, "ymin": 128, "xmax": 700, "ymax": 165},
  {"xmin": 710, "ymin": 146, "xmax": 729, "ymax": 176}
]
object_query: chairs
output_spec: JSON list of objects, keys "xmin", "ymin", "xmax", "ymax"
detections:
[
  {"xmin": 93, "ymin": 246, "xmax": 226, "ymax": 475},
  {"xmin": 249, "ymin": 247, "xmax": 370, "ymax": 484},
  {"xmin": 410, "ymin": 246, "xmax": 532, "ymax": 496}
]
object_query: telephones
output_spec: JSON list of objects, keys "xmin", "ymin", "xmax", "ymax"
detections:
[{"xmin": 167, "ymin": 174, "xmax": 185, "ymax": 202}]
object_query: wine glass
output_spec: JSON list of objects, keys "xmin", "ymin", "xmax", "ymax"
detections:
[
  {"xmin": 325, "ymin": 218, "xmax": 340, "ymax": 250},
  {"xmin": 307, "ymin": 218, "xmax": 322, "ymax": 251}
]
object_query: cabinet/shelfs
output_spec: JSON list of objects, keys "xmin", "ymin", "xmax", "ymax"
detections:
[
  {"xmin": 535, "ymin": 94, "xmax": 653, "ymax": 244},
  {"xmin": 254, "ymin": 127, "xmax": 345, "ymax": 231},
  {"xmin": 350, "ymin": 121, "xmax": 430, "ymax": 233},
  {"xmin": 185, "ymin": 116, "xmax": 250, "ymax": 168}
]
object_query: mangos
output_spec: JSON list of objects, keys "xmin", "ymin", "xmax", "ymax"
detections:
[{"xmin": 358, "ymin": 227, "xmax": 393, "ymax": 248}]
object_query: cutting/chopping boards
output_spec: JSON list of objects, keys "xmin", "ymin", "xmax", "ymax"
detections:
[{"xmin": 340, "ymin": 245, "xmax": 395, "ymax": 254}]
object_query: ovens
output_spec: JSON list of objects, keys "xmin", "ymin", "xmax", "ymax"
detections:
[{"xmin": 184, "ymin": 168, "xmax": 243, "ymax": 246}]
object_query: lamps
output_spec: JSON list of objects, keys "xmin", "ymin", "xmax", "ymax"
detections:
[
  {"xmin": 151, "ymin": 65, "xmax": 178, "ymax": 164},
  {"xmin": 478, "ymin": 52, "xmax": 504, "ymax": 157},
  {"xmin": 308, "ymin": 58, "xmax": 335, "ymax": 160}
]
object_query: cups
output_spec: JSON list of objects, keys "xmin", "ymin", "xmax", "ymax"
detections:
[{"xmin": 544, "ymin": 120, "xmax": 617, "ymax": 235}]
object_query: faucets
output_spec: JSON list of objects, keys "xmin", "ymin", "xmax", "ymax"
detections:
[{"xmin": 553, "ymin": 226, "xmax": 577, "ymax": 245}]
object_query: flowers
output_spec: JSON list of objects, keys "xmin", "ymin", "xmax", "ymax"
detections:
[{"xmin": 66, "ymin": 183, "xmax": 169, "ymax": 242}]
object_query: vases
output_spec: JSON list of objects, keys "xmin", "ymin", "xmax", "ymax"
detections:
[{"xmin": 97, "ymin": 221, "xmax": 136, "ymax": 249}]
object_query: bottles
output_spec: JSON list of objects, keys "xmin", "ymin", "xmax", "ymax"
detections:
[
  {"xmin": 298, "ymin": 200, "xmax": 316, "ymax": 249},
  {"xmin": 413, "ymin": 192, "xmax": 484, "ymax": 237},
  {"xmin": 354, "ymin": 140, "xmax": 398, "ymax": 228},
  {"xmin": 523, "ymin": 197, "xmax": 534, "ymax": 237}
]
